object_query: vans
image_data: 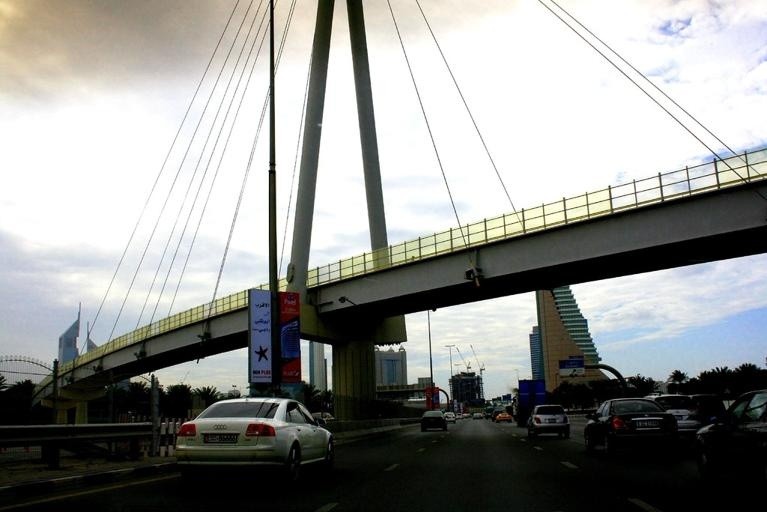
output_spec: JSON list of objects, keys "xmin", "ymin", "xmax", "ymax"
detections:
[{"xmin": 312, "ymin": 412, "xmax": 336, "ymax": 425}]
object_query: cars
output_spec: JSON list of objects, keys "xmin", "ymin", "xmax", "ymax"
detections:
[
  {"xmin": 420, "ymin": 411, "xmax": 448, "ymax": 433},
  {"xmin": 443, "ymin": 411, "xmax": 470, "ymax": 424},
  {"xmin": 525, "ymin": 404, "xmax": 572, "ymax": 439},
  {"xmin": 585, "ymin": 390, "xmax": 767, "ymax": 492},
  {"xmin": 471, "ymin": 406, "xmax": 515, "ymax": 424},
  {"xmin": 173, "ymin": 396, "xmax": 339, "ymax": 479}
]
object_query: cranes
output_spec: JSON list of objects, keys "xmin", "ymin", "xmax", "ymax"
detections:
[
  {"xmin": 455, "ymin": 347, "xmax": 471, "ymax": 376},
  {"xmin": 469, "ymin": 343, "xmax": 487, "ymax": 376}
]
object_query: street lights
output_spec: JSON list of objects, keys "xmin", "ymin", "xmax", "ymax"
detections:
[
  {"xmin": 444, "ymin": 342, "xmax": 456, "ymax": 379},
  {"xmin": 231, "ymin": 383, "xmax": 238, "ymax": 399},
  {"xmin": 426, "ymin": 306, "xmax": 440, "ymax": 413}
]
